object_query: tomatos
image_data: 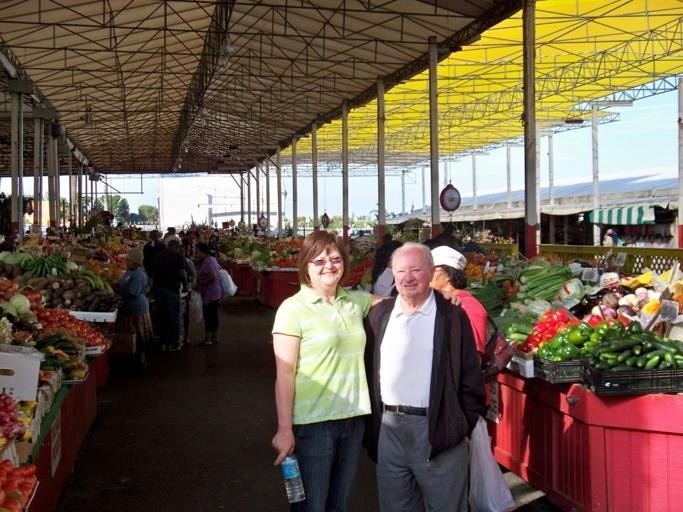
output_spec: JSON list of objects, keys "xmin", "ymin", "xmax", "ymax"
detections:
[
  {"xmin": 0, "ymin": 459, "xmax": 37, "ymax": 512},
  {"xmin": 37, "ymin": 308, "xmax": 111, "ymax": 349}
]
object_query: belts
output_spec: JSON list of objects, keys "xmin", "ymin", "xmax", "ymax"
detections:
[{"xmin": 383, "ymin": 404, "xmax": 426, "ymax": 417}]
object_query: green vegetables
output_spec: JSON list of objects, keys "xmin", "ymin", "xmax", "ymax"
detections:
[{"xmin": 524, "ymin": 263, "xmax": 574, "ymax": 299}]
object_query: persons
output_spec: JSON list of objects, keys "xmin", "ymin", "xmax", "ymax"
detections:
[
  {"xmin": 367, "ymin": 239, "xmax": 401, "ymax": 298},
  {"xmin": 250, "ymin": 224, "xmax": 258, "ymax": 234},
  {"xmin": 360, "ymin": 241, "xmax": 488, "ymax": 511},
  {"xmin": 600, "ymin": 228, "xmax": 617, "ymax": 247},
  {"xmin": 427, "ymin": 244, "xmax": 518, "ymax": 511},
  {"xmin": 110, "ymin": 226, "xmax": 225, "ymax": 372},
  {"xmin": 266, "ymin": 227, "xmax": 465, "ymax": 511}
]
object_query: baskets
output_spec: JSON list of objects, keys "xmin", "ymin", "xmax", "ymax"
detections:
[
  {"xmin": 580, "ymin": 359, "xmax": 683, "ymax": 395},
  {"xmin": 531, "ymin": 356, "xmax": 585, "ymax": 385}
]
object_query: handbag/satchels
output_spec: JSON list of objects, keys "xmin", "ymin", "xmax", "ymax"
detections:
[
  {"xmin": 481, "ymin": 331, "xmax": 513, "ymax": 384},
  {"xmin": 219, "ymin": 269, "xmax": 238, "ymax": 296}
]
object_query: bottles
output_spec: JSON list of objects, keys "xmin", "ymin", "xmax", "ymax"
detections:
[{"xmin": 281, "ymin": 452, "xmax": 306, "ymax": 504}]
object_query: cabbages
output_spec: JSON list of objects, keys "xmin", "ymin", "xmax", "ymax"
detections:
[{"xmin": 0, "ymin": 294, "xmax": 39, "ymax": 326}]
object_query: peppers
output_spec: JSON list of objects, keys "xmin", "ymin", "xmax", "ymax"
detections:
[{"xmin": 536, "ymin": 321, "xmax": 608, "ymax": 362}]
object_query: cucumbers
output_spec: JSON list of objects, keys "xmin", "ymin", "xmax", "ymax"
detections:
[{"xmin": 586, "ymin": 321, "xmax": 683, "ymax": 371}]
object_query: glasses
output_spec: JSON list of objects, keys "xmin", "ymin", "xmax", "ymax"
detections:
[{"xmin": 309, "ymin": 257, "xmax": 345, "ymax": 266}]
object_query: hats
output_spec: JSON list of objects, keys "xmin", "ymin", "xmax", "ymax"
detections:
[
  {"xmin": 127, "ymin": 248, "xmax": 144, "ymax": 265},
  {"xmin": 431, "ymin": 246, "xmax": 468, "ymax": 271},
  {"xmin": 164, "ymin": 236, "xmax": 182, "ymax": 248}
]
